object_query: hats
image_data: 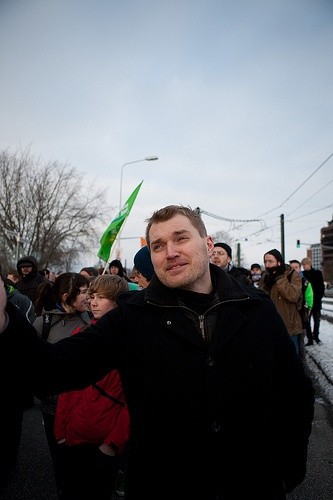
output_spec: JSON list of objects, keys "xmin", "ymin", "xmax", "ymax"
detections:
[
  {"xmin": 108, "ymin": 259, "xmax": 124, "ymax": 278},
  {"xmin": 134, "ymin": 245, "xmax": 156, "ymax": 282},
  {"xmin": 214, "ymin": 242, "xmax": 232, "ymax": 258},
  {"xmin": 250, "ymin": 263, "xmax": 261, "ymax": 270},
  {"xmin": 19, "ymin": 261, "xmax": 32, "ymax": 268},
  {"xmin": 263, "ymin": 249, "xmax": 284, "ymax": 265}
]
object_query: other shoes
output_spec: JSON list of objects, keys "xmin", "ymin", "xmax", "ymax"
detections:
[
  {"xmin": 314, "ymin": 337, "xmax": 321, "ymax": 344},
  {"xmin": 305, "ymin": 341, "xmax": 314, "ymax": 346}
]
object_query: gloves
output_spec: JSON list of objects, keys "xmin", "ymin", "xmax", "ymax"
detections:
[{"xmin": 270, "ymin": 267, "xmax": 280, "ymax": 277}]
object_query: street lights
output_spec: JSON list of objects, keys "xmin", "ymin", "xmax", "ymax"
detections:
[{"xmin": 116, "ymin": 157, "xmax": 159, "ymax": 261}]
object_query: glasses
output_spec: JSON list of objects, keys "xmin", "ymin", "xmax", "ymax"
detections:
[{"xmin": 212, "ymin": 252, "xmax": 224, "ymax": 255}]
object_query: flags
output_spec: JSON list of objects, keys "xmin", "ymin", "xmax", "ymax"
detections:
[{"xmin": 97, "ymin": 186, "xmax": 142, "ymax": 260}]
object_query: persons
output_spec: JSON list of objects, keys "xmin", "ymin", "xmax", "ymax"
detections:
[
  {"xmin": 0, "ymin": 236, "xmax": 326, "ymax": 500},
  {"xmin": 0, "ymin": 207, "xmax": 313, "ymax": 500}
]
